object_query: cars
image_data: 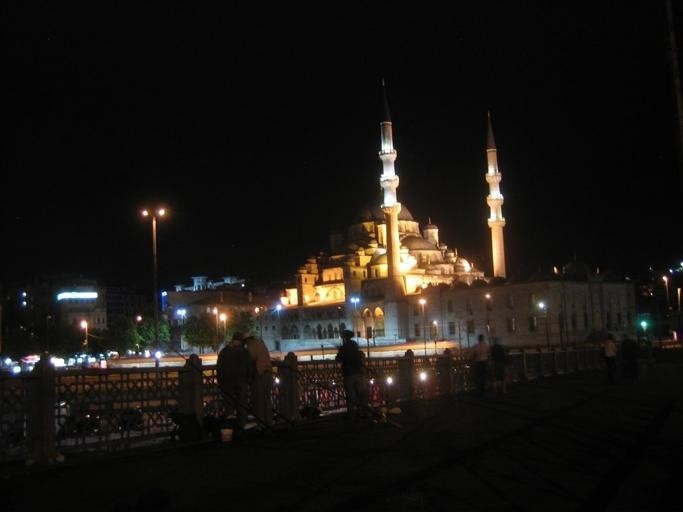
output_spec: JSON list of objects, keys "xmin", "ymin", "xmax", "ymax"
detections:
[{"xmin": 0, "ymin": 346, "xmax": 213, "ymax": 375}]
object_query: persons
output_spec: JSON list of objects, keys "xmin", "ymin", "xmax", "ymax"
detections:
[
  {"xmin": 489, "ymin": 338, "xmax": 502, "ymax": 379},
  {"xmin": 242, "ymin": 332, "xmax": 273, "ymax": 429},
  {"xmin": 469, "ymin": 335, "xmax": 489, "ymax": 377},
  {"xmin": 334, "ymin": 330, "xmax": 365, "ymax": 413},
  {"xmin": 603, "ymin": 335, "xmax": 615, "ymax": 365},
  {"xmin": 217, "ymin": 332, "xmax": 257, "ymax": 440}
]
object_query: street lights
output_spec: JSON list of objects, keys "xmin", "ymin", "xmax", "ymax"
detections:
[
  {"xmin": 253, "ymin": 306, "xmax": 263, "ymax": 340},
  {"xmin": 213, "ymin": 307, "xmax": 219, "ymax": 347},
  {"xmin": 177, "ymin": 308, "xmax": 187, "ymax": 360},
  {"xmin": 221, "ymin": 313, "xmax": 228, "ymax": 338},
  {"xmin": 80, "ymin": 319, "xmax": 89, "ymax": 356},
  {"xmin": 139, "ymin": 206, "xmax": 168, "ymax": 369},
  {"xmin": 335, "ymin": 303, "xmax": 344, "ymax": 337}
]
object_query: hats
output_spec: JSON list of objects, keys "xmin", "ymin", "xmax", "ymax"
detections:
[
  {"xmin": 242, "ymin": 332, "xmax": 250, "ymax": 340},
  {"xmin": 233, "ymin": 331, "xmax": 241, "ymax": 340}
]
